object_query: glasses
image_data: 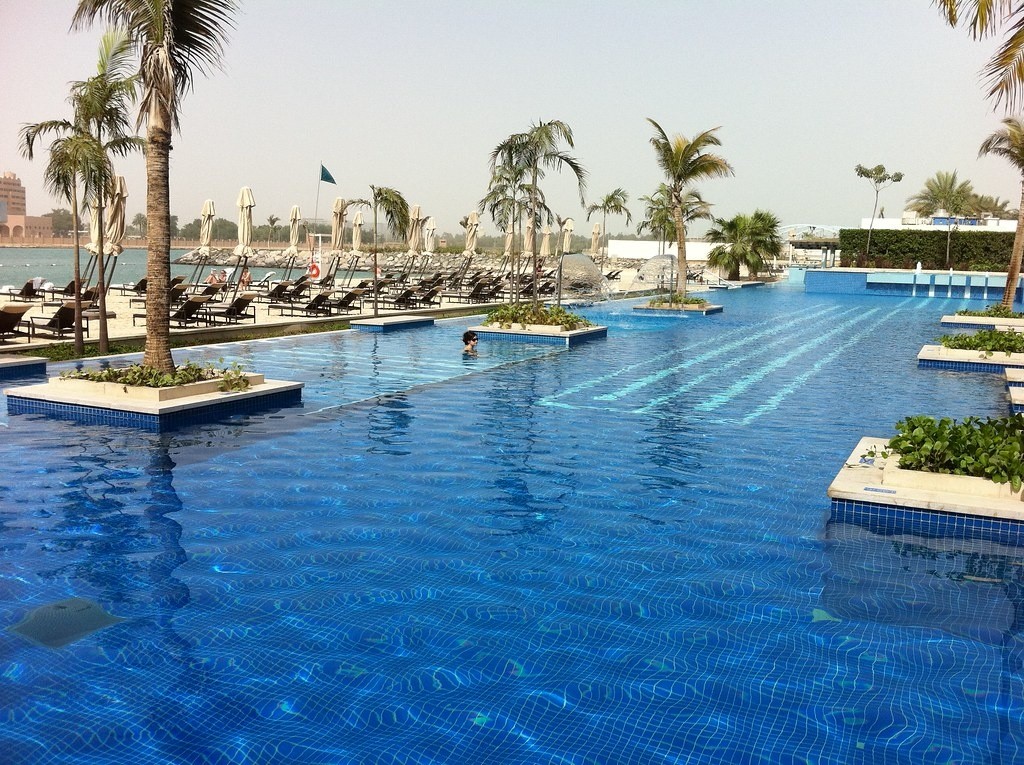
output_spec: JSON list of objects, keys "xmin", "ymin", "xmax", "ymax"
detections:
[{"xmin": 469, "ymin": 337, "xmax": 479, "ymax": 342}]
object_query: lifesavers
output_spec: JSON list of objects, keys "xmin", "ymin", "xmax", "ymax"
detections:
[{"xmin": 309, "ymin": 265, "xmax": 320, "ymax": 278}]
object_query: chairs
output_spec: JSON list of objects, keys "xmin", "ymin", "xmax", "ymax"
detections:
[{"xmin": 0, "ymin": 269, "xmax": 705, "ymax": 345}]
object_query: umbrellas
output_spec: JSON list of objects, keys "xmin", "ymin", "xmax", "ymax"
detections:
[
  {"xmin": 563, "ymin": 219, "xmax": 574, "ymax": 254},
  {"xmin": 225, "ymin": 187, "xmax": 256, "ymax": 304},
  {"xmin": 413, "ymin": 218, "xmax": 437, "ymax": 283},
  {"xmin": 321, "ymin": 198, "xmax": 348, "ymax": 293},
  {"xmin": 81, "ymin": 180, "xmax": 106, "ymax": 293},
  {"xmin": 281, "ymin": 205, "xmax": 301, "ymax": 281},
  {"xmin": 188, "ymin": 199, "xmax": 215, "ymax": 292},
  {"xmin": 391, "ymin": 206, "xmax": 423, "ymax": 294},
  {"xmin": 519, "ymin": 218, "xmax": 532, "ymax": 280},
  {"xmin": 340, "ymin": 211, "xmax": 365, "ymax": 288},
  {"xmin": 91, "ymin": 175, "xmax": 129, "ymax": 306},
  {"xmin": 497, "ymin": 224, "xmax": 512, "ymax": 276},
  {"xmin": 450, "ymin": 212, "xmax": 480, "ymax": 290},
  {"xmin": 591, "ymin": 223, "xmax": 601, "ymax": 262},
  {"xmin": 540, "ymin": 226, "xmax": 552, "ymax": 266}
]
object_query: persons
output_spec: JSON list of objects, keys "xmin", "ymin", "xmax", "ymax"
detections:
[
  {"xmin": 205, "ymin": 271, "xmax": 228, "ymax": 292},
  {"xmin": 241, "ymin": 267, "xmax": 251, "ymax": 291},
  {"xmin": 537, "ymin": 259, "xmax": 542, "ymax": 271},
  {"xmin": 463, "ymin": 331, "xmax": 479, "ymax": 351},
  {"xmin": 377, "ymin": 266, "xmax": 381, "ymax": 276}
]
